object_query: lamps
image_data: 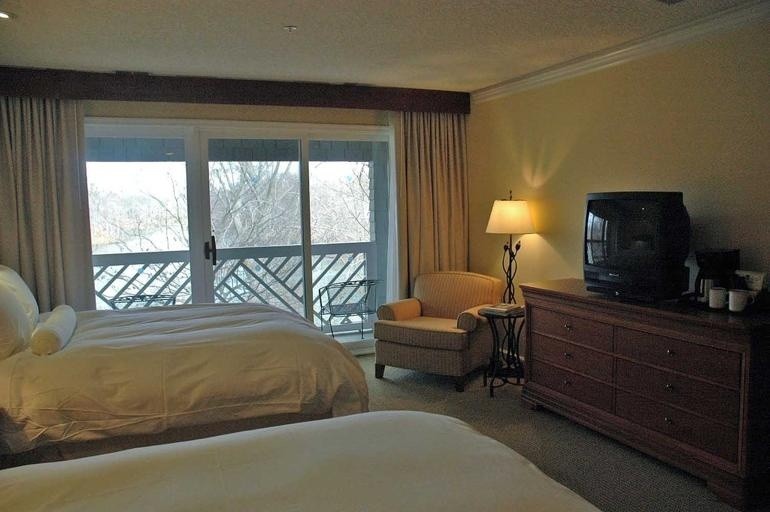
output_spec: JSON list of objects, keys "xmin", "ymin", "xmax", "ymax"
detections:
[{"xmin": 484, "ymin": 190, "xmax": 540, "ymax": 376}]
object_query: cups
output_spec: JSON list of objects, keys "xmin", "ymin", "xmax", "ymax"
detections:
[{"xmin": 709, "ymin": 287, "xmax": 755, "ymax": 312}]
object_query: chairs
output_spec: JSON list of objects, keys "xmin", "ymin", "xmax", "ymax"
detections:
[{"xmin": 318, "ymin": 271, "xmax": 504, "ymax": 393}]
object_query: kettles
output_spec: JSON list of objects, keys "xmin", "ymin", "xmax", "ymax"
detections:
[{"xmin": 695, "ymin": 273, "xmax": 718, "ymax": 309}]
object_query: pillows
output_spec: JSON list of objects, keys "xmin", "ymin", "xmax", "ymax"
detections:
[
  {"xmin": 30, "ymin": 304, "xmax": 77, "ymax": 356},
  {"xmin": 1, "ymin": 261, "xmax": 40, "ymax": 334}
]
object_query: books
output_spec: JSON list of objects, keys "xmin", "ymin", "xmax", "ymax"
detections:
[{"xmin": 480, "ymin": 303, "xmax": 521, "ymax": 317}]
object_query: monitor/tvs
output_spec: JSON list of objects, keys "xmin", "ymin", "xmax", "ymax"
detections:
[{"xmin": 582, "ymin": 191, "xmax": 691, "ymax": 308}]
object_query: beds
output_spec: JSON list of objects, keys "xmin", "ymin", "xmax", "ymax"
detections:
[
  {"xmin": 1, "ymin": 303, "xmax": 369, "ymax": 467},
  {"xmin": 0, "ymin": 410, "xmax": 602, "ymax": 512}
]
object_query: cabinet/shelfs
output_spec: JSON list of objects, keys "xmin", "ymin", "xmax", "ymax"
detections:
[{"xmin": 517, "ymin": 278, "xmax": 770, "ymax": 512}]
